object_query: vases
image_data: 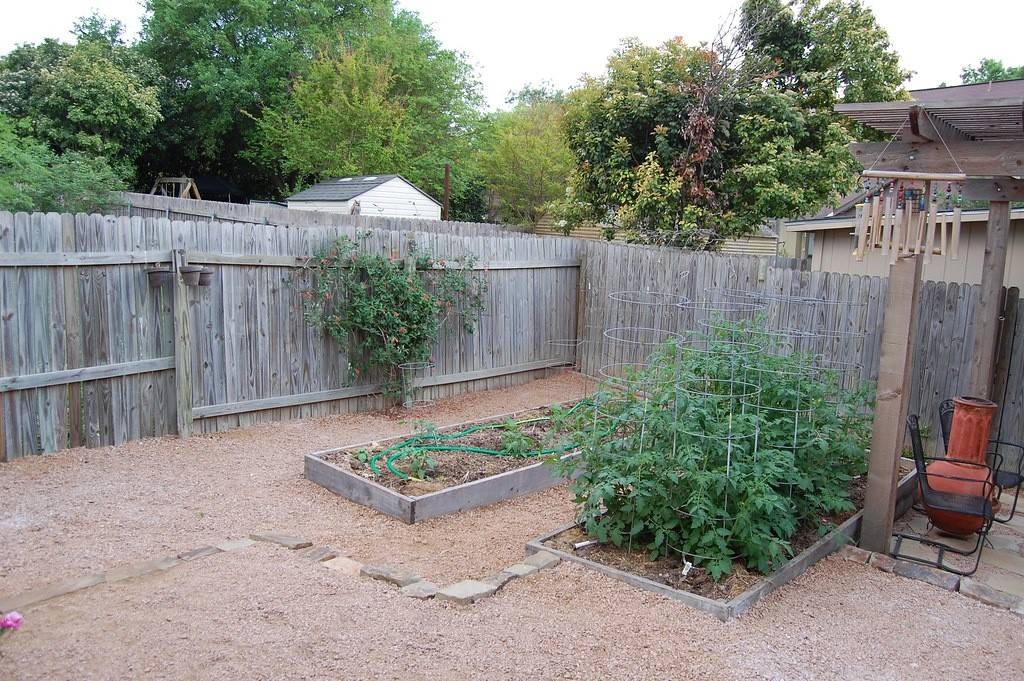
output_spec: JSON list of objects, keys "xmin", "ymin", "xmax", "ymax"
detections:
[
  {"xmin": 917, "ymin": 396, "xmax": 1002, "ymax": 541},
  {"xmin": 146, "ymin": 267, "xmax": 169, "ymax": 287},
  {"xmin": 180, "ymin": 266, "xmax": 202, "ymax": 286},
  {"xmin": 199, "ymin": 269, "xmax": 216, "ymax": 286}
]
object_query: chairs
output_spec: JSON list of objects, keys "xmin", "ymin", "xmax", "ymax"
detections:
[{"xmin": 890, "ymin": 399, "xmax": 1024, "ymax": 576}]
